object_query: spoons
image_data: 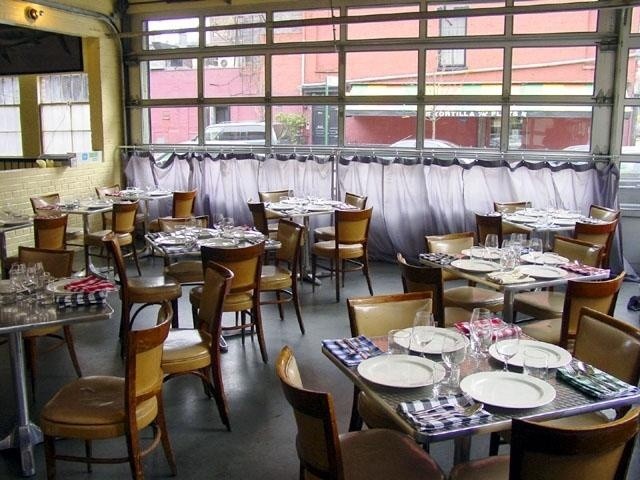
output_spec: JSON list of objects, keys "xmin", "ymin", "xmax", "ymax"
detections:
[
  {"xmin": 577, "ymin": 361, "xmax": 626, "ymax": 388},
  {"xmin": 420, "ymin": 403, "xmax": 484, "ymax": 423}
]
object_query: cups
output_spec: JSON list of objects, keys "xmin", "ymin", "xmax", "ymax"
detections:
[
  {"xmin": 279, "ymin": 195, "xmax": 288, "ymax": 207},
  {"xmin": 387, "ymin": 329, "xmax": 411, "ymax": 355}
]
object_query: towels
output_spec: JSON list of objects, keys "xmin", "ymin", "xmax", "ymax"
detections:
[
  {"xmin": 558, "ymin": 364, "xmax": 639, "ymax": 402},
  {"xmin": 324, "ymin": 335, "xmax": 383, "ymax": 365},
  {"xmin": 398, "ymin": 394, "xmax": 493, "ymax": 433}
]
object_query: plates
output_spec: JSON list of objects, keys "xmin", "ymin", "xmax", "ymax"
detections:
[
  {"xmin": 506, "ymin": 208, "xmax": 582, "ymax": 226},
  {"xmin": 459, "ymin": 370, "xmax": 557, "ymax": 409},
  {"xmin": 46, "ymin": 277, "xmax": 85, "ymax": 294},
  {"xmin": 270, "ymin": 199, "xmax": 349, "ymax": 211},
  {"xmin": 154, "ymin": 228, "xmax": 261, "ymax": 247},
  {"xmin": 488, "ymin": 339, "xmax": 572, "ymax": 369},
  {"xmin": 450, "ymin": 245, "xmax": 570, "ymax": 279},
  {"xmin": 56, "ymin": 189, "xmax": 167, "ymax": 208},
  {"xmin": 357, "ymin": 353, "xmax": 446, "ymax": 388},
  {"xmin": 394, "ymin": 326, "xmax": 470, "ymax": 354}
]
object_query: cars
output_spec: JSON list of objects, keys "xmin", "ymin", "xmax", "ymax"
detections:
[{"xmin": 387, "ymin": 137, "xmax": 460, "ymax": 149}]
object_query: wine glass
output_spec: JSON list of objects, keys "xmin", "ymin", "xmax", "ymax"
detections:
[
  {"xmin": 525, "ymin": 197, "xmax": 572, "ymax": 224},
  {"xmin": 484, "ymin": 232, "xmax": 543, "ymax": 271},
  {"xmin": 287, "ymin": 185, "xmax": 320, "ymax": 210},
  {"xmin": 186, "ymin": 213, "xmax": 244, "ymax": 247},
  {"xmin": 8, "ymin": 261, "xmax": 56, "ymax": 304},
  {"xmin": 413, "ymin": 308, "xmax": 518, "ymax": 397},
  {"xmin": 1, "ymin": 299, "xmax": 57, "ymax": 325}
]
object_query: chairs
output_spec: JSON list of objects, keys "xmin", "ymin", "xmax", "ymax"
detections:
[
  {"xmin": 152, "ymin": 258, "xmax": 234, "ymax": 435},
  {"xmin": 489, "ymin": 308, "xmax": 640, "ymax": 456},
  {"xmin": 278, "ymin": 346, "xmax": 447, "ymax": 480},
  {"xmin": 450, "ymin": 404, "xmax": 640, "ymax": 480},
  {"xmin": 40, "ymin": 301, "xmax": 178, "ymax": 479},
  {"xmin": 347, "ymin": 289, "xmax": 434, "ymax": 455}
]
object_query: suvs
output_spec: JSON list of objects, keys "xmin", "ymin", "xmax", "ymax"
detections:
[{"xmin": 178, "ymin": 121, "xmax": 293, "ymax": 145}]
